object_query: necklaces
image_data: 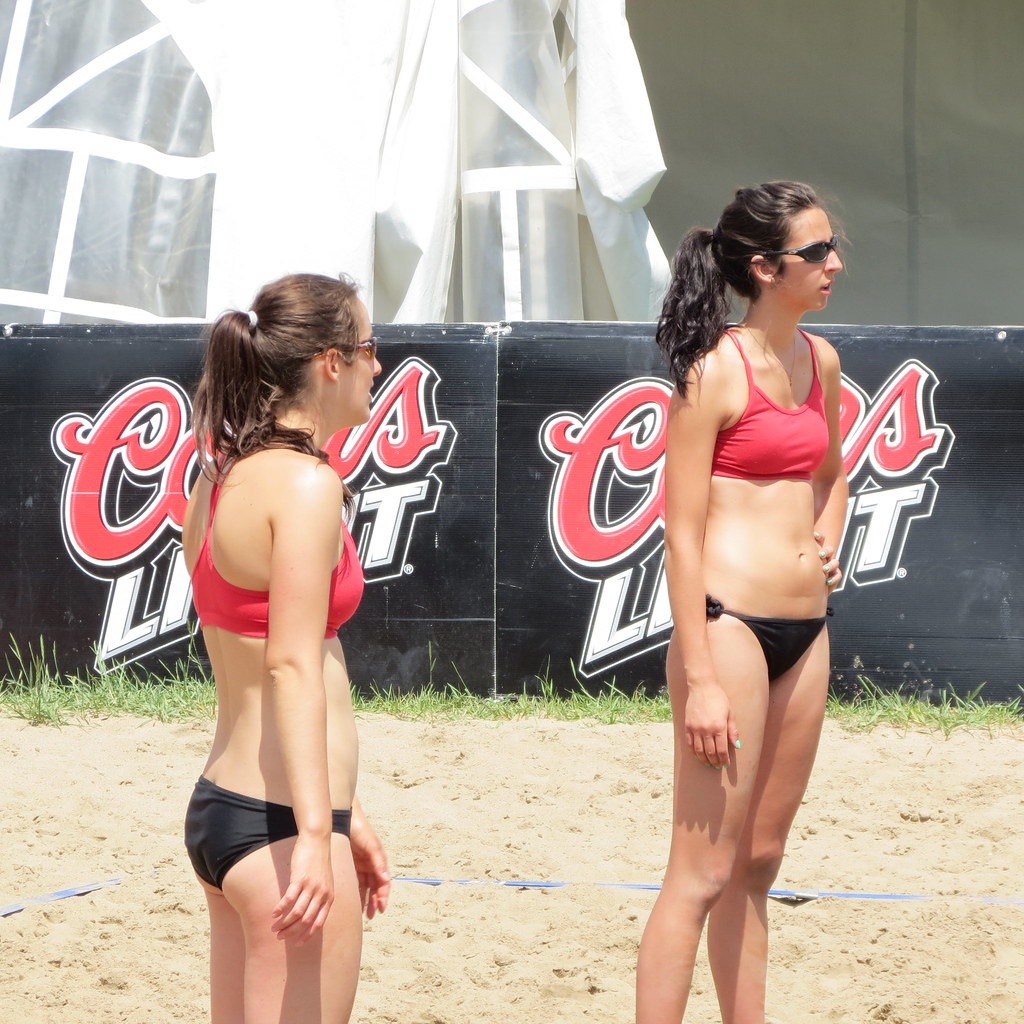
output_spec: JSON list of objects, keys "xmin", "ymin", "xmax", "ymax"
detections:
[{"xmin": 745, "ymin": 319, "xmax": 797, "ymax": 386}]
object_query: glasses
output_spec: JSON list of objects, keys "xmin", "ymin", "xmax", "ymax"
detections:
[
  {"xmin": 303, "ymin": 336, "xmax": 377, "ymax": 363},
  {"xmin": 759, "ymin": 233, "xmax": 838, "ymax": 263}
]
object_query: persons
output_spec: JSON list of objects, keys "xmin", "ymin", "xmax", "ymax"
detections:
[
  {"xmin": 635, "ymin": 182, "xmax": 850, "ymax": 1024},
  {"xmin": 181, "ymin": 271, "xmax": 391, "ymax": 1024}
]
